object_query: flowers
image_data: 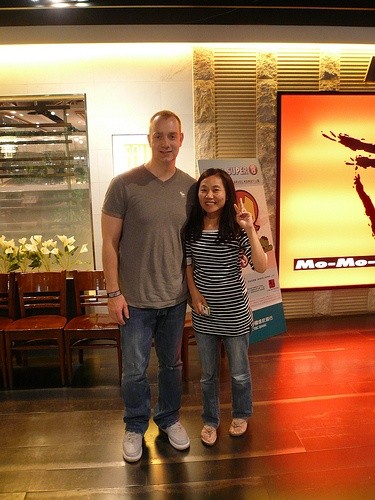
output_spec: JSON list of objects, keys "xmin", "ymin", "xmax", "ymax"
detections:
[{"xmin": 0, "ymin": 234, "xmax": 92, "ymax": 277}]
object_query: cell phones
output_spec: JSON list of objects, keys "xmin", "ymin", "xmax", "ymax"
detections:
[{"xmin": 201, "ymin": 305, "xmax": 210, "ymax": 316}]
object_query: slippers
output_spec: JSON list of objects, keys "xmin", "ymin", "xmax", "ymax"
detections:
[
  {"xmin": 229, "ymin": 418, "xmax": 248, "ymax": 437},
  {"xmin": 201, "ymin": 426, "xmax": 217, "ymax": 446}
]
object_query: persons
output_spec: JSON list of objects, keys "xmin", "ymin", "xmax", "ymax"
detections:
[
  {"xmin": 102, "ymin": 110, "xmax": 249, "ymax": 462},
  {"xmin": 183, "ymin": 168, "xmax": 267, "ymax": 445}
]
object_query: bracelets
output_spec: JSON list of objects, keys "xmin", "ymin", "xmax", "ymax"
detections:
[{"xmin": 107, "ymin": 290, "xmax": 121, "ymax": 298}]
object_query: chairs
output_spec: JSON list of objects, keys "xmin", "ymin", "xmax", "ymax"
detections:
[
  {"xmin": 0, "ymin": 270, "xmax": 123, "ymax": 389},
  {"xmin": 182, "ymin": 312, "xmax": 226, "ymax": 383}
]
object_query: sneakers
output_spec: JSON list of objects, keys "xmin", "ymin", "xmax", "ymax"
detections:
[
  {"xmin": 161, "ymin": 422, "xmax": 190, "ymax": 450},
  {"xmin": 124, "ymin": 431, "xmax": 142, "ymax": 462}
]
